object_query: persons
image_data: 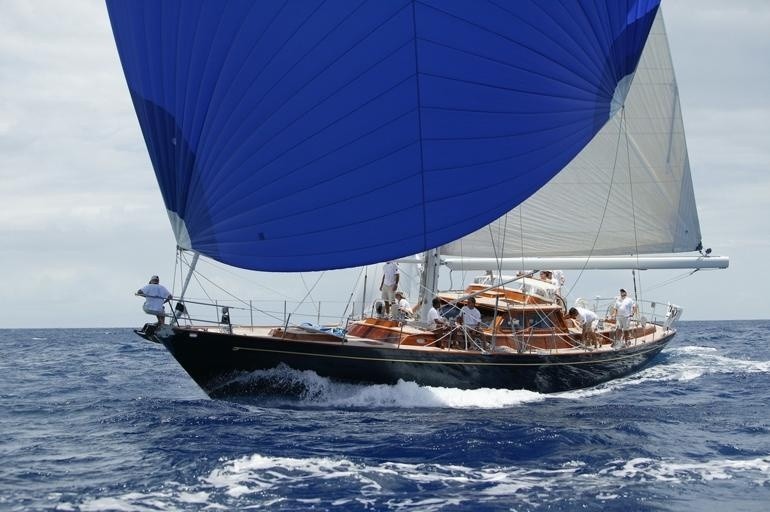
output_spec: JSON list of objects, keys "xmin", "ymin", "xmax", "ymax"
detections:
[
  {"xmin": 547, "ymin": 272, "xmax": 558, "ymax": 285},
  {"xmin": 539, "ymin": 271, "xmax": 552, "ymax": 284},
  {"xmin": 454, "ymin": 297, "xmax": 487, "ymax": 350},
  {"xmin": 379, "ymin": 260, "xmax": 399, "ymax": 317},
  {"xmin": 611, "ymin": 289, "xmax": 637, "ymax": 348},
  {"xmin": 557, "ymin": 307, "xmax": 600, "ymax": 349},
  {"xmin": 138, "ymin": 274, "xmax": 173, "ymax": 325},
  {"xmin": 428, "ymin": 297, "xmax": 445, "ymax": 349},
  {"xmin": 396, "ymin": 291, "xmax": 412, "ymax": 319}
]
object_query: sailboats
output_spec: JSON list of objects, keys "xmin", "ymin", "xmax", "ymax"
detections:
[{"xmin": 105, "ymin": 0, "xmax": 729, "ymax": 401}]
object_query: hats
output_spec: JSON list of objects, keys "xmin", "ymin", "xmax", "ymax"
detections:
[
  {"xmin": 149, "ymin": 276, "xmax": 159, "ymax": 284},
  {"xmin": 433, "ymin": 299, "xmax": 440, "ymax": 304},
  {"xmin": 396, "ymin": 292, "xmax": 405, "ymax": 298},
  {"xmin": 620, "ymin": 288, "xmax": 628, "ymax": 295}
]
